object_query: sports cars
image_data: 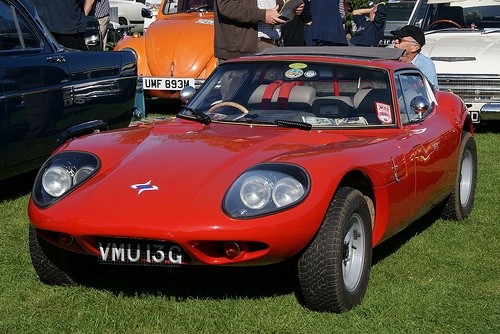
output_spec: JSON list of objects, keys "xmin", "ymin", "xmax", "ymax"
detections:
[{"xmin": 27, "ymin": 45, "xmax": 478, "ymax": 313}]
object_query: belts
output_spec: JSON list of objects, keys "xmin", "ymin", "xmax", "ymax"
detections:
[{"xmin": 99, "ymin": 13, "xmax": 110, "ymax": 18}]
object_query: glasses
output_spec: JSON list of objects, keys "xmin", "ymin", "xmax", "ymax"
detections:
[{"xmin": 394, "ymin": 37, "xmax": 416, "ymax": 44}]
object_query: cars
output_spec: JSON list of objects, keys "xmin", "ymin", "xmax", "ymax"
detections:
[
  {"xmin": 111, "ymin": 0, "xmax": 220, "ymax": 103},
  {"xmin": 106, "ymin": 0, "xmax": 179, "ymax": 37},
  {"xmin": 0, "ymin": 0, "xmax": 137, "ymax": 187},
  {"xmin": 386, "ymin": 0, "xmax": 500, "ymax": 128},
  {"xmin": 378, "ymin": 0, "xmax": 418, "ymax": 47}
]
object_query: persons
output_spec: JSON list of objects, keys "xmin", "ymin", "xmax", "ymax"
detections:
[
  {"xmin": 389, "ymin": 25, "xmax": 440, "ymax": 91},
  {"xmin": 211, "ymin": 0, "xmax": 305, "ymax": 103},
  {"xmin": 346, "ymin": 2, "xmax": 387, "ymax": 47},
  {"xmin": 84, "ymin": 0, "xmax": 111, "ymax": 40},
  {"xmin": 301, "ymin": 0, "xmax": 348, "ymax": 45}
]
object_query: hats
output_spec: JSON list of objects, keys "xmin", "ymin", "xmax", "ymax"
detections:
[{"xmin": 390, "ymin": 25, "xmax": 425, "ymax": 47}]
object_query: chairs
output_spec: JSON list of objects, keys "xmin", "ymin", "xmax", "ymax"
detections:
[
  {"xmin": 353, "ymin": 87, "xmax": 418, "ymax": 125},
  {"xmin": 247, "ymin": 79, "xmax": 316, "ymax": 113}
]
